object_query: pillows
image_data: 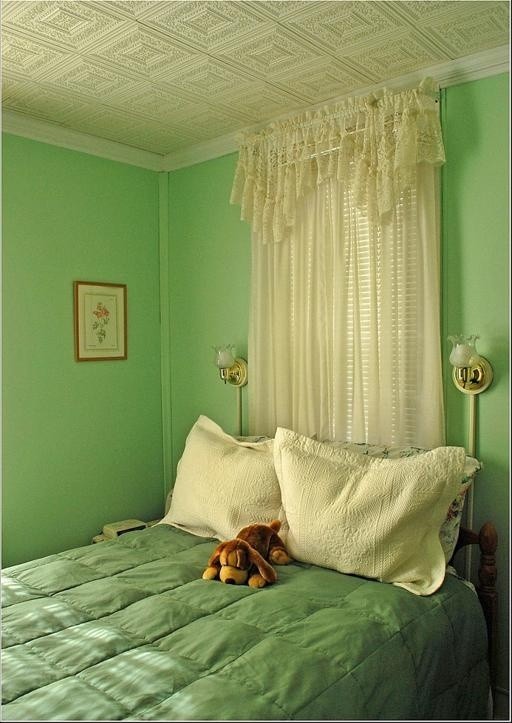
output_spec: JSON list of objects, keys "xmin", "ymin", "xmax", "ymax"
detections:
[
  {"xmin": 273, "ymin": 427, "xmax": 467, "ymax": 598},
  {"xmin": 311, "ymin": 434, "xmax": 482, "ymax": 568},
  {"xmin": 153, "ymin": 416, "xmax": 280, "ymax": 548}
]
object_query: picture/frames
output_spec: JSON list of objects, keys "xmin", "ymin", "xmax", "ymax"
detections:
[{"xmin": 75, "ymin": 282, "xmax": 126, "ymax": 361}]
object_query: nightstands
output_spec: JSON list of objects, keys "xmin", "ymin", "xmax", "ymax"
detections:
[{"xmin": 92, "ymin": 518, "xmax": 162, "ymax": 544}]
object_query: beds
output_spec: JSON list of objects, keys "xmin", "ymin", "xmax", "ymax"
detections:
[{"xmin": 1, "ymin": 521, "xmax": 497, "ymax": 720}]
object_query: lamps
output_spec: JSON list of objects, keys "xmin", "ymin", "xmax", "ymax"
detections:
[
  {"xmin": 212, "ymin": 344, "xmax": 247, "ymax": 437},
  {"xmin": 447, "ymin": 334, "xmax": 494, "ymax": 580}
]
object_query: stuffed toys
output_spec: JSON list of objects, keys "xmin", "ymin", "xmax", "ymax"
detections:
[{"xmin": 202, "ymin": 520, "xmax": 290, "ymax": 588}]
